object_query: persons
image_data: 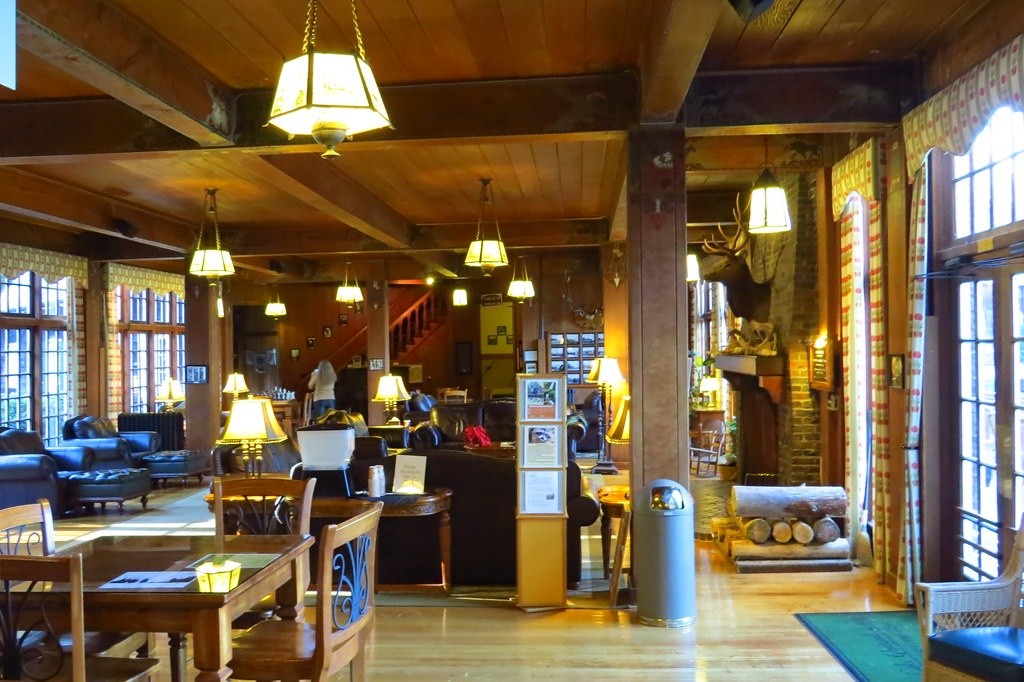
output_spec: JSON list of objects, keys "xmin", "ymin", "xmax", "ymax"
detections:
[{"xmin": 308, "ymin": 360, "xmax": 337, "ymax": 416}]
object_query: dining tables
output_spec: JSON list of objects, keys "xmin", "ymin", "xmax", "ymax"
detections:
[{"xmin": 214, "ymin": 372, "xmax": 288, "ymax": 477}]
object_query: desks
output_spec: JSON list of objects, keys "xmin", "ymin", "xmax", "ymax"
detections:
[
  {"xmin": 202, "ymin": 485, "xmax": 454, "ymax": 598},
  {"xmin": 0, "ymin": 533, "xmax": 317, "ymax": 682},
  {"xmin": 688, "ymin": 407, "xmax": 727, "ymax": 459}
]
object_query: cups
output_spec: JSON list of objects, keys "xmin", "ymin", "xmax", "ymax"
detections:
[{"xmin": 368, "ymin": 465, "xmax": 386, "ymax": 498}]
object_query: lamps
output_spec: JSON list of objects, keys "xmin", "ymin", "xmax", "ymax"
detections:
[
  {"xmin": 746, "ymin": 135, "xmax": 792, "ymax": 235},
  {"xmin": 584, "ymin": 356, "xmax": 626, "ymax": 474},
  {"xmin": 370, "ymin": 374, "xmax": 411, "ymax": 425},
  {"xmin": 335, "ymin": 262, "xmax": 364, "ymax": 308},
  {"xmin": 195, "ymin": 553, "xmax": 242, "ymax": 592},
  {"xmin": 507, "ymin": 255, "xmax": 535, "ymax": 305},
  {"xmin": 700, "ymin": 377, "xmax": 720, "ymax": 406},
  {"xmin": 261, "ymin": 0, "xmax": 395, "ymax": 159},
  {"xmin": 264, "ymin": 284, "xmax": 288, "ymax": 321},
  {"xmin": 464, "ymin": 176, "xmax": 509, "ymax": 277},
  {"xmin": 189, "ymin": 187, "xmax": 236, "ymax": 287}
]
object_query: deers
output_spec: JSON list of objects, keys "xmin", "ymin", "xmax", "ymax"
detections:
[
  {"xmin": 701, "ymin": 181, "xmax": 772, "ymax": 322},
  {"xmin": 728, "ymin": 319, "xmax": 777, "ymax": 355}
]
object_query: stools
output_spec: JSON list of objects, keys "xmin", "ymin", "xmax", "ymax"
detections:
[
  {"xmin": 143, "ymin": 448, "xmax": 210, "ymax": 487},
  {"xmin": 66, "ymin": 465, "xmax": 150, "ymax": 514}
]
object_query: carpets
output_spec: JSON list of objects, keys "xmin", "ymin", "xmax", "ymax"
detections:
[{"xmin": 795, "ymin": 608, "xmax": 923, "ymax": 682}]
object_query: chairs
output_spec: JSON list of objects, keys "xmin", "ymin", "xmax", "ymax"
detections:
[
  {"xmin": 0, "ymin": 499, "xmax": 384, "ymax": 682},
  {"xmin": 913, "ymin": 511, "xmax": 1024, "ymax": 682}
]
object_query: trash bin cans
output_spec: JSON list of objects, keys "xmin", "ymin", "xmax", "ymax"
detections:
[{"xmin": 632, "ymin": 479, "xmax": 697, "ymax": 627}]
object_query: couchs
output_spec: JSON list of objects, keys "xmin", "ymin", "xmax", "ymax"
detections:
[
  {"xmin": 63, "ymin": 415, "xmax": 162, "ymax": 468},
  {"xmin": 0, "ymin": 427, "xmax": 98, "ymax": 520},
  {"xmin": 298, "ymin": 390, "xmax": 601, "ymax": 589}
]
